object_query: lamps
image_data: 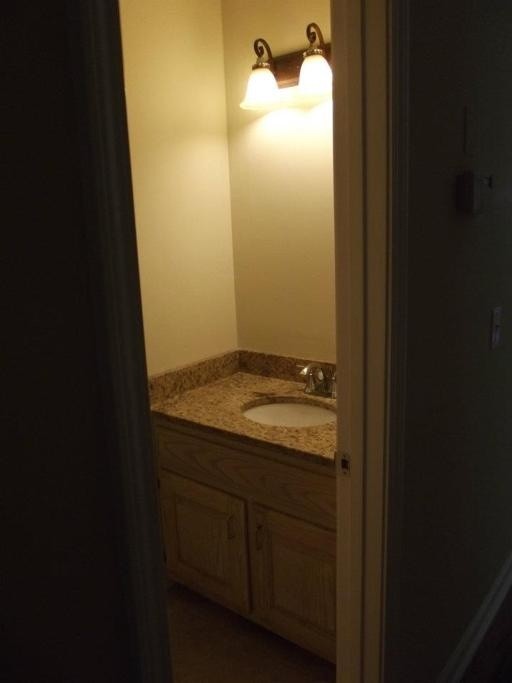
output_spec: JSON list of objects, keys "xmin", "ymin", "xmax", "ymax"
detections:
[{"xmin": 237, "ymin": 22, "xmax": 332, "ymax": 113}]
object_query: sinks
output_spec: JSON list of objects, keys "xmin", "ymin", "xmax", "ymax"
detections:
[{"xmin": 240, "ymin": 395, "xmax": 338, "ymax": 429}]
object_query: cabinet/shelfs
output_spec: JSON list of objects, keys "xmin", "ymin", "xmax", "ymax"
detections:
[{"xmin": 153, "ymin": 424, "xmax": 336, "ymax": 663}]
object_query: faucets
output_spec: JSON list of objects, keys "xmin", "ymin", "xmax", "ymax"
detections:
[{"xmin": 298, "ymin": 362, "xmax": 335, "ymax": 395}]
